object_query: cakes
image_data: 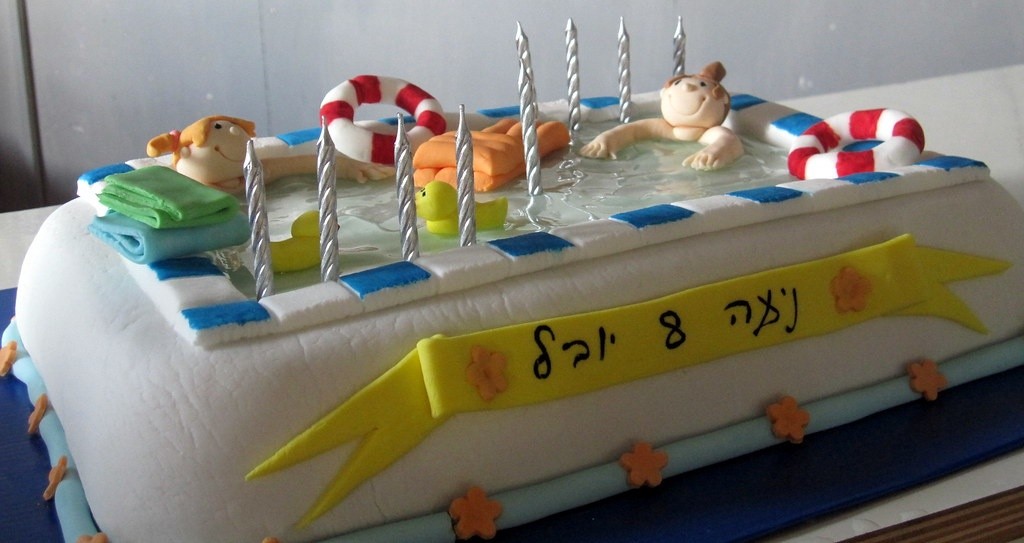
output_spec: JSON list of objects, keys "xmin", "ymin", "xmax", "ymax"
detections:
[{"xmin": 0, "ymin": 58, "xmax": 1024, "ymax": 543}]
